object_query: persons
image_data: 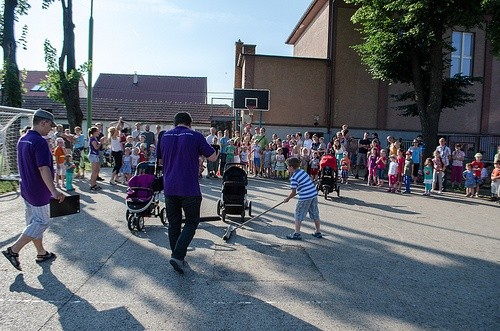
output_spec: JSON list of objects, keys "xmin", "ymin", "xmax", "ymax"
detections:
[
  {"xmin": 1, "ymin": 109, "xmax": 66, "ymax": 270},
  {"xmin": 126, "ymin": 161, "xmax": 159, "ymax": 212},
  {"xmin": 284, "ymin": 157, "xmax": 323, "ymax": 240},
  {"xmin": 155, "ymin": 111, "xmax": 219, "ymax": 275},
  {"xmin": 19, "ymin": 117, "xmax": 500, "ymax": 205}
]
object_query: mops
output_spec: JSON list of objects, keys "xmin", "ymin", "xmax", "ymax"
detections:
[{"xmin": 222, "ymin": 192, "xmax": 300, "ymax": 240}]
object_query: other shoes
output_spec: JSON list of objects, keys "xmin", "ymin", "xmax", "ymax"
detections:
[
  {"xmin": 68, "ymin": 188, "xmax": 75, "ymax": 192},
  {"xmin": 97, "ymin": 177, "xmax": 104, "ymax": 181},
  {"xmin": 423, "ymin": 186, "xmax": 500, "ymax": 201},
  {"xmin": 90, "ymin": 184, "xmax": 103, "ymax": 192},
  {"xmin": 249, "ymin": 169, "xmax": 281, "ymax": 179},
  {"xmin": 169, "ymin": 258, "xmax": 186, "ymax": 274},
  {"xmin": 212, "ymin": 173, "xmax": 218, "ymax": 178},
  {"xmin": 386, "ymin": 189, "xmax": 409, "ymax": 193},
  {"xmin": 115, "ymin": 180, "xmax": 123, "ymax": 184},
  {"xmin": 55, "ymin": 184, "xmax": 61, "ymax": 189},
  {"xmin": 110, "ymin": 182, "xmax": 117, "ymax": 186},
  {"xmin": 207, "ymin": 174, "xmax": 212, "ymax": 178}
]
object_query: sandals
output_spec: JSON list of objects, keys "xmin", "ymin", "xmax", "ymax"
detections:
[
  {"xmin": 2, "ymin": 247, "xmax": 25, "ymax": 272},
  {"xmin": 36, "ymin": 251, "xmax": 55, "ymax": 263}
]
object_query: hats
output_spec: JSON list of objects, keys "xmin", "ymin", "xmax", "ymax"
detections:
[
  {"xmin": 35, "ymin": 109, "xmax": 57, "ymax": 128},
  {"xmin": 474, "ymin": 153, "xmax": 482, "ymax": 158},
  {"xmin": 404, "ymin": 151, "xmax": 411, "ymax": 155}
]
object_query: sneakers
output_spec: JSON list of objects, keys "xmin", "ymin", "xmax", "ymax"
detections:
[
  {"xmin": 312, "ymin": 232, "xmax": 322, "ymax": 238},
  {"xmin": 286, "ymin": 233, "xmax": 301, "ymax": 240}
]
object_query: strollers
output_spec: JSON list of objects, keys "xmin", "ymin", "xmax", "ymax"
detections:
[
  {"xmin": 316, "ymin": 155, "xmax": 340, "ymax": 199},
  {"xmin": 217, "ymin": 162, "xmax": 252, "ymax": 222},
  {"xmin": 126, "ymin": 162, "xmax": 166, "ymax": 231}
]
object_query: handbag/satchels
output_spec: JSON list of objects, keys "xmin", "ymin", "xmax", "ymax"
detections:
[{"xmin": 151, "ymin": 176, "xmax": 164, "ymax": 191}]
